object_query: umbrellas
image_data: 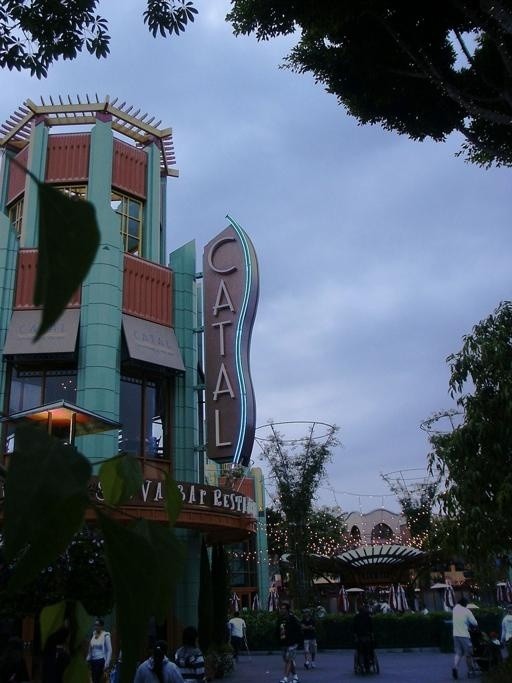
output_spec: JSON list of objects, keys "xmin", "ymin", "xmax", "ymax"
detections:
[
  {"xmin": 230, "ymin": 592, "xmax": 241, "ymax": 611},
  {"xmin": 394, "ymin": 583, "xmax": 409, "ymax": 612},
  {"xmin": 497, "ymin": 577, "xmax": 512, "ymax": 602},
  {"xmin": 429, "ymin": 581, "xmax": 452, "ymax": 589},
  {"xmin": 268, "ymin": 588, "xmax": 279, "ymax": 611},
  {"xmin": 388, "ymin": 582, "xmax": 397, "ymax": 612},
  {"xmin": 250, "ymin": 591, "xmax": 261, "ymax": 610},
  {"xmin": 443, "ymin": 578, "xmax": 457, "ymax": 611},
  {"xmin": 338, "ymin": 583, "xmax": 348, "ymax": 612}
]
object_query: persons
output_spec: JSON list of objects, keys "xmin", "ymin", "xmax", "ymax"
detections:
[
  {"xmin": 275, "ymin": 603, "xmax": 301, "ymax": 682},
  {"xmin": 362, "ymin": 597, "xmax": 390, "ymax": 614},
  {"xmin": 84, "ymin": 616, "xmax": 112, "ymax": 682},
  {"xmin": 174, "ymin": 625, "xmax": 208, "ymax": 682},
  {"xmin": 353, "ymin": 610, "xmax": 375, "ymax": 674},
  {"xmin": 301, "ymin": 607, "xmax": 318, "ymax": 669},
  {"xmin": 451, "ymin": 596, "xmax": 478, "ymax": 679},
  {"xmin": 500, "ymin": 602, "xmax": 512, "ymax": 665},
  {"xmin": 135, "ymin": 638, "xmax": 186, "ymax": 682},
  {"xmin": 228, "ymin": 610, "xmax": 247, "ymax": 661},
  {"xmin": 490, "ymin": 630, "xmax": 500, "ymax": 645}
]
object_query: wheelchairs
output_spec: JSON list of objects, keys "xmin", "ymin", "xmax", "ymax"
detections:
[{"xmin": 352, "ymin": 649, "xmax": 381, "ymax": 676}]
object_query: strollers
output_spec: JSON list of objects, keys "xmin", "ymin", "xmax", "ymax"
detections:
[{"xmin": 466, "ymin": 630, "xmax": 504, "ymax": 678}]
